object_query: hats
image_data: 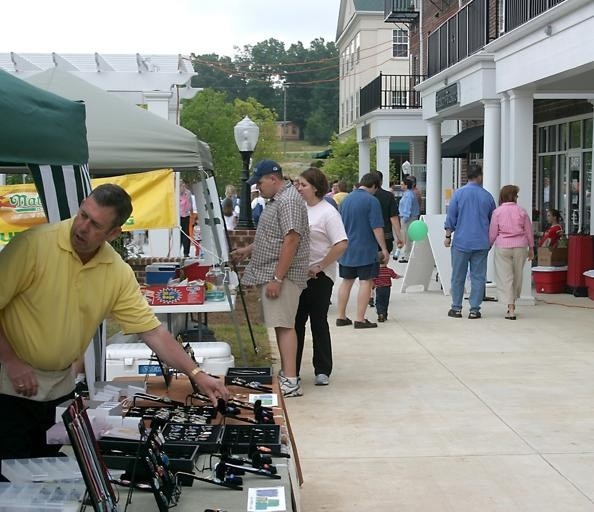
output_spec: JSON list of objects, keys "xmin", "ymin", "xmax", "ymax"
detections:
[
  {"xmin": 246, "ymin": 160, "xmax": 280, "ymax": 186},
  {"xmin": 250, "ymin": 184, "xmax": 259, "ymax": 192}
]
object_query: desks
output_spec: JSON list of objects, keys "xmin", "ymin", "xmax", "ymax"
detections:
[
  {"xmin": 148, "ymin": 287, "xmax": 238, "ymax": 343},
  {"xmin": 71, "ymin": 375, "xmax": 305, "ymax": 512}
]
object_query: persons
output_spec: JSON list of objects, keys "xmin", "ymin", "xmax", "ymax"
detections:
[
  {"xmin": 372, "ymin": 251, "xmax": 404, "ymax": 322},
  {"xmin": 229, "ymin": 161, "xmax": 310, "ymax": 397},
  {"xmin": 222, "ymin": 185, "xmax": 237, "ymax": 209},
  {"xmin": 388, "ymin": 181, "xmax": 395, "ymax": 195},
  {"xmin": 333, "ymin": 181, "xmax": 349, "ymax": 204},
  {"xmin": 444, "ymin": 164, "xmax": 496, "ymax": 318},
  {"xmin": 538, "ymin": 208, "xmax": 563, "ymax": 248},
  {"xmin": 336, "ymin": 173, "xmax": 390, "ymax": 329},
  {"xmin": 324, "ymin": 183, "xmax": 337, "ymax": 198},
  {"xmin": 250, "ymin": 184, "xmax": 265, "ymax": 223},
  {"xmin": 294, "ymin": 167, "xmax": 349, "ymax": 387},
  {"xmin": 368, "ymin": 170, "xmax": 404, "ymax": 307},
  {"xmin": 488, "ymin": 184, "xmax": 535, "ymax": 320},
  {"xmin": 0, "ymin": 183, "xmax": 231, "ymax": 487},
  {"xmin": 398, "ymin": 177, "xmax": 420, "ymax": 263},
  {"xmin": 223, "ymin": 199, "xmax": 237, "ymax": 231},
  {"xmin": 407, "ymin": 176, "xmax": 422, "ymax": 217},
  {"xmin": 179, "ymin": 178, "xmax": 193, "ymax": 257}
]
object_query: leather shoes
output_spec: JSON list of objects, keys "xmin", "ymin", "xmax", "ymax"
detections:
[
  {"xmin": 448, "ymin": 309, "xmax": 462, "ymax": 317},
  {"xmin": 354, "ymin": 319, "xmax": 377, "ymax": 328},
  {"xmin": 336, "ymin": 317, "xmax": 353, "ymax": 326},
  {"xmin": 468, "ymin": 311, "xmax": 481, "ymax": 318}
]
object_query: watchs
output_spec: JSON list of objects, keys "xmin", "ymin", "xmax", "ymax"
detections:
[{"xmin": 271, "ymin": 274, "xmax": 282, "ymax": 284}]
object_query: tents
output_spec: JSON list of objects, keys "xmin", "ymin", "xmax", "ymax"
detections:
[
  {"xmin": 0, "ymin": 63, "xmax": 109, "ymax": 385},
  {"xmin": 20, "ymin": 65, "xmax": 258, "ymax": 361}
]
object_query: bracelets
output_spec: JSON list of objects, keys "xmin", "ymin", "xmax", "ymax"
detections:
[{"xmin": 189, "ymin": 366, "xmax": 203, "ymax": 379}]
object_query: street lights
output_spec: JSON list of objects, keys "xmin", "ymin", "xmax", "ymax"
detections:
[{"xmin": 234, "ymin": 115, "xmax": 259, "ymax": 229}]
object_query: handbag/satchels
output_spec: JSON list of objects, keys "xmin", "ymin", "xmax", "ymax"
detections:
[{"xmin": 251, "ymin": 198, "xmax": 263, "ymax": 223}]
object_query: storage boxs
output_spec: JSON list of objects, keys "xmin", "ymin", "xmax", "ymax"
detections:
[
  {"xmin": 566, "ymin": 233, "xmax": 594, "ymax": 296},
  {"xmin": 532, "ymin": 265, "xmax": 567, "ymax": 294},
  {"xmin": 107, "ymin": 341, "xmax": 234, "ymax": 383},
  {"xmin": 136, "ymin": 281, "xmax": 206, "ymax": 305},
  {"xmin": 583, "ymin": 270, "xmax": 594, "ymax": 301}
]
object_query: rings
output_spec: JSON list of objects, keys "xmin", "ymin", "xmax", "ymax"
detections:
[{"xmin": 16, "ymin": 384, "xmax": 25, "ymax": 388}]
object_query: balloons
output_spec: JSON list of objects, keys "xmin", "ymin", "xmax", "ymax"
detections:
[{"xmin": 408, "ymin": 217, "xmax": 429, "ymax": 242}]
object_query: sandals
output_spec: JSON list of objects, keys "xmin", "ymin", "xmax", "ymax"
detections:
[{"xmin": 505, "ymin": 310, "xmax": 516, "ymax": 320}]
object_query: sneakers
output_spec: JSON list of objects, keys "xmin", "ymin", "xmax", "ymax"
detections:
[
  {"xmin": 280, "ymin": 377, "xmax": 303, "ymax": 397},
  {"xmin": 399, "ymin": 258, "xmax": 408, "ymax": 263},
  {"xmin": 278, "ymin": 368, "xmax": 302, "ymax": 388},
  {"xmin": 314, "ymin": 374, "xmax": 329, "ymax": 385},
  {"xmin": 379, "ymin": 314, "xmax": 384, "ymax": 322}
]
